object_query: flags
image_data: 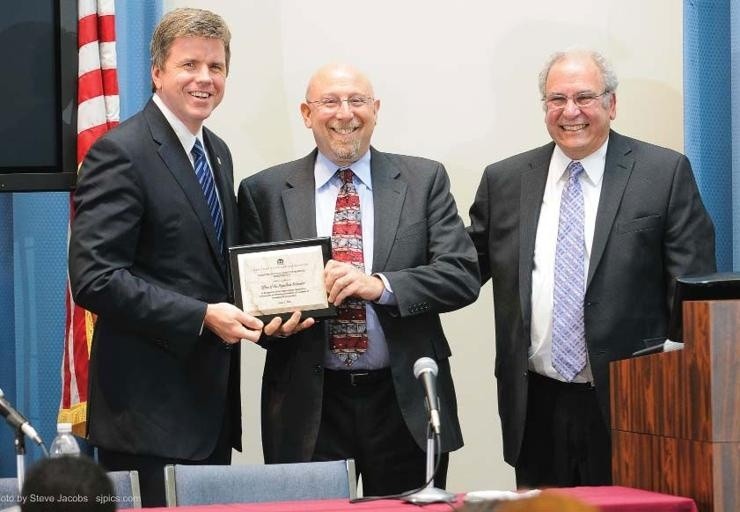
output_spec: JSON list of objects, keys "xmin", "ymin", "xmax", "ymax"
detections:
[{"xmin": 54, "ymin": 1, "xmax": 121, "ymax": 444}]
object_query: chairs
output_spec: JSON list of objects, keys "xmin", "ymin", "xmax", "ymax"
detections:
[
  {"xmin": 0, "ymin": 470, "xmax": 142, "ymax": 510},
  {"xmin": 163, "ymin": 460, "xmax": 359, "ymax": 503}
]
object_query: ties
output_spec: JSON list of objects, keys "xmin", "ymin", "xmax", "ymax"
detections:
[
  {"xmin": 328, "ymin": 168, "xmax": 368, "ymax": 367},
  {"xmin": 191, "ymin": 138, "xmax": 224, "ymax": 255},
  {"xmin": 551, "ymin": 159, "xmax": 587, "ymax": 384}
]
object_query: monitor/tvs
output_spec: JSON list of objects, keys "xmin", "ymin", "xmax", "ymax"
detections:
[{"xmin": 0, "ymin": 0, "xmax": 80, "ymax": 193}]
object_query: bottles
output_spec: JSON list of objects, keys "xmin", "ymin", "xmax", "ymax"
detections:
[{"xmin": 48, "ymin": 419, "xmax": 82, "ymax": 458}]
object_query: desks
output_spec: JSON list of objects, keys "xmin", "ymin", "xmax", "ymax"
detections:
[{"xmin": 118, "ymin": 485, "xmax": 695, "ymax": 510}]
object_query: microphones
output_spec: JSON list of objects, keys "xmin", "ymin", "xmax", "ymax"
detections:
[
  {"xmin": 0, "ymin": 388, "xmax": 44, "ymax": 445},
  {"xmin": 413, "ymin": 357, "xmax": 441, "ymax": 434}
]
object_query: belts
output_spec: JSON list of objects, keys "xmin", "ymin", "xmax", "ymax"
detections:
[{"xmin": 325, "ymin": 369, "xmax": 392, "ymax": 388}]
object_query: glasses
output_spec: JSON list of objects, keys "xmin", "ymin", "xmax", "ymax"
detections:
[
  {"xmin": 308, "ymin": 96, "xmax": 376, "ymax": 109},
  {"xmin": 541, "ymin": 91, "xmax": 608, "ymax": 108}
]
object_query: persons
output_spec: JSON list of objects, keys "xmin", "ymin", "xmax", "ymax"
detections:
[
  {"xmin": 463, "ymin": 46, "xmax": 717, "ymax": 491},
  {"xmin": 18, "ymin": 451, "xmax": 118, "ymax": 512},
  {"xmin": 66, "ymin": 6, "xmax": 265, "ymax": 508},
  {"xmin": 235, "ymin": 58, "xmax": 483, "ymax": 501}
]
